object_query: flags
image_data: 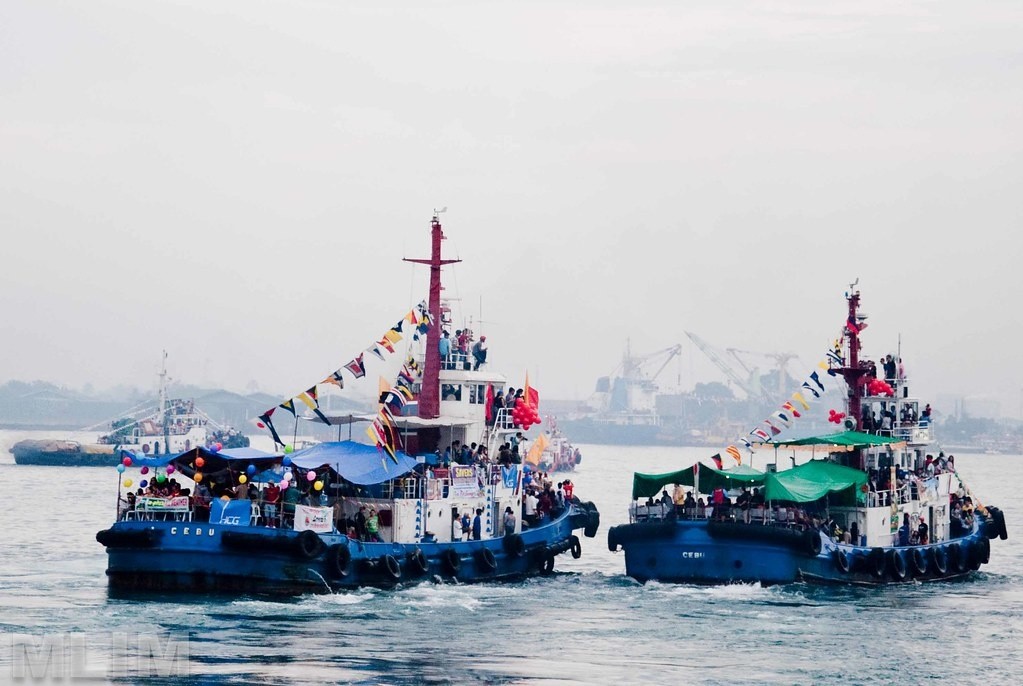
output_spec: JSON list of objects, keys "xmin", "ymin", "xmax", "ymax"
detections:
[
  {"xmin": 691, "ymin": 392, "xmax": 810, "ymax": 477},
  {"xmin": 249, "ymin": 298, "xmax": 435, "ymax": 473},
  {"xmin": 802, "ymin": 315, "xmax": 859, "ymax": 398}
]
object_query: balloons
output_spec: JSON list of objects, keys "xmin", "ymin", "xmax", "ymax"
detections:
[
  {"xmin": 868, "ymin": 379, "xmax": 894, "ymax": 396},
  {"xmin": 512, "ymin": 398, "xmax": 542, "ymax": 430},
  {"xmin": 829, "ymin": 409, "xmax": 846, "ymax": 424},
  {"xmin": 116, "ymin": 442, "xmax": 323, "ymax": 490}
]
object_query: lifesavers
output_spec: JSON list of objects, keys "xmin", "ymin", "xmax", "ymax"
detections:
[
  {"xmin": 297, "ymin": 530, "xmax": 321, "ymax": 558},
  {"xmin": 571, "ymin": 536, "xmax": 581, "ymax": 559},
  {"xmin": 444, "ymin": 548, "xmax": 462, "ymax": 572},
  {"xmin": 416, "ymin": 549, "xmax": 429, "ymax": 572},
  {"xmin": 907, "ymin": 548, "xmax": 925, "ymax": 575},
  {"xmin": 972, "ymin": 505, "xmax": 1008, "ymax": 570},
  {"xmin": 384, "ymin": 554, "xmax": 401, "ymax": 578},
  {"xmin": 835, "ymin": 550, "xmax": 849, "ymax": 573},
  {"xmin": 803, "ymin": 529, "xmax": 822, "ymax": 555},
  {"xmin": 887, "ymin": 549, "xmax": 906, "ymax": 579},
  {"xmin": 329, "ymin": 544, "xmax": 351, "ymax": 577},
  {"xmin": 584, "ymin": 500, "xmax": 600, "ymax": 537},
  {"xmin": 508, "ymin": 534, "xmax": 525, "ymax": 557},
  {"xmin": 540, "ymin": 552, "xmax": 554, "ymax": 575},
  {"xmin": 931, "ymin": 547, "xmax": 946, "ymax": 574},
  {"xmin": 481, "ymin": 547, "xmax": 497, "ymax": 569}
]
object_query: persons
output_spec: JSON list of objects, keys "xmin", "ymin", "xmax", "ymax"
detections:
[
  {"xmin": 883, "ymin": 452, "xmax": 955, "ymax": 497},
  {"xmin": 493, "ymin": 387, "xmax": 523, "ymax": 429},
  {"xmin": 438, "ymin": 329, "xmax": 488, "ymax": 371},
  {"xmin": 898, "ymin": 516, "xmax": 928, "ymax": 546},
  {"xmin": 789, "ymin": 502, "xmax": 806, "ymax": 524},
  {"xmin": 836, "ymin": 521, "xmax": 859, "ymax": 546},
  {"xmin": 950, "ymin": 497, "xmax": 974, "ymax": 539},
  {"xmin": 453, "ymin": 506, "xmax": 483, "ymax": 542},
  {"xmin": 522, "ymin": 470, "xmax": 574, "ymax": 529},
  {"xmin": 880, "ymin": 355, "xmax": 909, "ymax": 399},
  {"xmin": 118, "ymin": 477, "xmax": 386, "ymax": 543},
  {"xmin": 444, "ymin": 432, "xmax": 524, "ymax": 469},
  {"xmin": 872, "ymin": 403, "xmax": 931, "ymax": 435},
  {"xmin": 563, "ymin": 441, "xmax": 579, "ymax": 459},
  {"xmin": 644, "ymin": 483, "xmax": 765, "ymax": 523},
  {"xmin": 503, "ymin": 506, "xmax": 517, "ymax": 535},
  {"xmin": 866, "ymin": 361, "xmax": 877, "ymax": 396}
]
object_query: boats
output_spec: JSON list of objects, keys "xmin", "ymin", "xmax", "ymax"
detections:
[
  {"xmin": 96, "ymin": 206, "xmax": 600, "ymax": 599},
  {"xmin": 606, "ymin": 276, "xmax": 1008, "ymax": 585},
  {"xmin": 7, "ymin": 350, "xmax": 250, "ymax": 465},
  {"xmin": 558, "ymin": 332, "xmax": 1023, "ymax": 452}
]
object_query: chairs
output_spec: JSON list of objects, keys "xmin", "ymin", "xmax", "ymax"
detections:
[
  {"xmin": 628, "ymin": 505, "xmax": 807, "ymax": 531},
  {"xmin": 125, "ymin": 495, "xmax": 262, "ymax": 527}
]
